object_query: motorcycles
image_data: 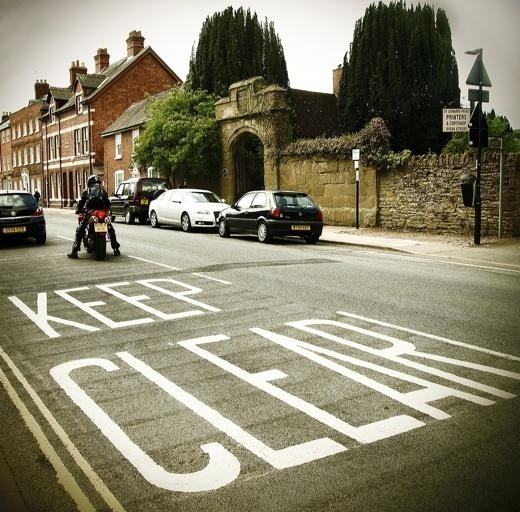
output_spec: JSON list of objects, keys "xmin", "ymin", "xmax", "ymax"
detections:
[{"xmin": 75, "ymin": 209, "xmax": 115, "ymax": 260}]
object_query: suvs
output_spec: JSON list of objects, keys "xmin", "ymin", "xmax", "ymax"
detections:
[
  {"xmin": 0, "ymin": 190, "xmax": 47, "ymax": 246},
  {"xmin": 107, "ymin": 177, "xmax": 174, "ymax": 223}
]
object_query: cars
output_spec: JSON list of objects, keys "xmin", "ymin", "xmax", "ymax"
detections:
[
  {"xmin": 147, "ymin": 187, "xmax": 232, "ymax": 233},
  {"xmin": 216, "ymin": 191, "xmax": 325, "ymax": 245}
]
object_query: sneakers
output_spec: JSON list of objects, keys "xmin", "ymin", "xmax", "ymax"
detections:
[
  {"xmin": 67, "ymin": 253, "xmax": 79, "ymax": 259},
  {"xmin": 112, "ymin": 248, "xmax": 120, "ymax": 256}
]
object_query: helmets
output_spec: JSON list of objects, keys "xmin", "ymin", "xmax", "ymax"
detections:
[{"xmin": 86, "ymin": 174, "xmax": 101, "ymax": 187}]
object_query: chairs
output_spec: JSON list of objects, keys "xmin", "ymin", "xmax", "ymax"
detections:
[{"xmin": 278, "ymin": 197, "xmax": 300, "ymax": 206}]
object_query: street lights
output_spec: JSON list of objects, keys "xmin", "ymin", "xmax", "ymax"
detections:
[{"xmin": 464, "ymin": 46, "xmax": 490, "ymax": 243}]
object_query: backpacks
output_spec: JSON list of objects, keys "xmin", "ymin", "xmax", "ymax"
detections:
[{"xmin": 85, "ymin": 187, "xmax": 111, "ymax": 209}]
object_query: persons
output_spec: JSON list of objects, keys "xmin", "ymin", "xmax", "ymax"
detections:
[
  {"xmin": 66, "ymin": 174, "xmax": 121, "ymax": 261},
  {"xmin": 34, "ymin": 188, "xmax": 40, "ymax": 203}
]
